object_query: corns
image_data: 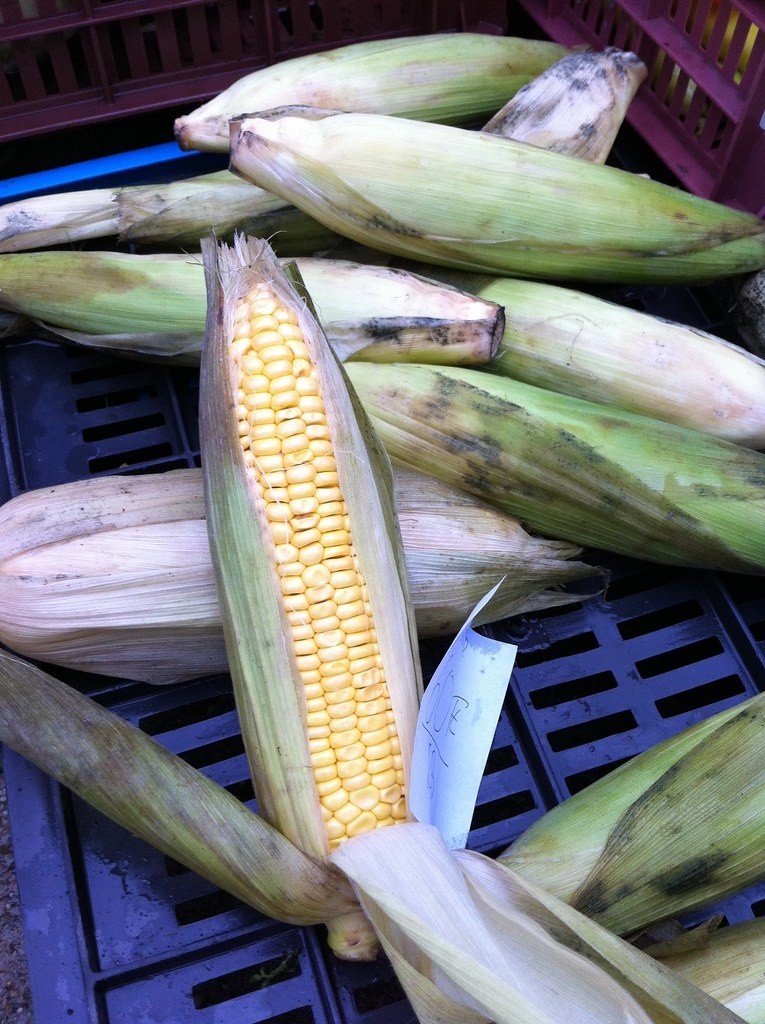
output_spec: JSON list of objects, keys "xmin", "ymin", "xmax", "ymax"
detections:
[{"xmin": 0, "ymin": 36, "xmax": 765, "ymax": 1024}]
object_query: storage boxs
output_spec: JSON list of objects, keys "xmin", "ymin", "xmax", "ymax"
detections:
[{"xmin": 0, "ymin": 0, "xmax": 765, "ymax": 1024}]
